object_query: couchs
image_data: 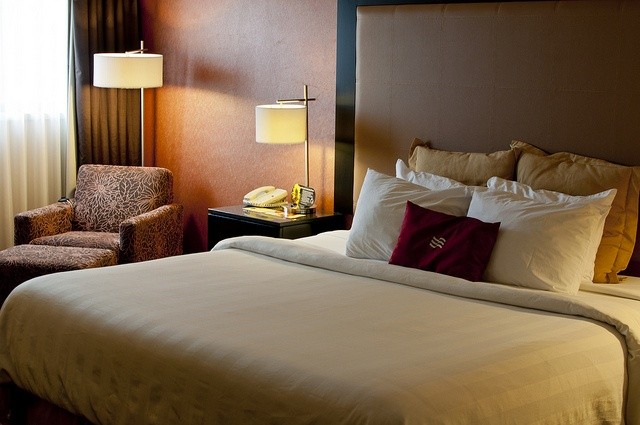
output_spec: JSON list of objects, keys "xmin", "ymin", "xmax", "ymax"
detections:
[{"xmin": 1, "ymin": 164, "xmax": 184, "ymax": 293}]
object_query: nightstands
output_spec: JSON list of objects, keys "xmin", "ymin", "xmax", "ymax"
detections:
[{"xmin": 207, "ymin": 204, "xmax": 344, "ymax": 254}]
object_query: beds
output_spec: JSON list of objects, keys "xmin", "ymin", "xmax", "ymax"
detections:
[{"xmin": 0, "ymin": 227, "xmax": 640, "ymax": 425}]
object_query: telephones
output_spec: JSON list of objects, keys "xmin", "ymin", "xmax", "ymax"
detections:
[{"xmin": 243, "ymin": 185, "xmax": 289, "ymax": 208}]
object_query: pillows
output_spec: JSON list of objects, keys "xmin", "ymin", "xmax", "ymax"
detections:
[
  {"xmin": 347, "ymin": 168, "xmax": 472, "ymax": 267},
  {"xmin": 486, "ymin": 175, "xmax": 617, "ymax": 290},
  {"xmin": 509, "ymin": 139, "xmax": 640, "ymax": 285},
  {"xmin": 387, "ymin": 201, "xmax": 499, "ymax": 280},
  {"xmin": 393, "ymin": 157, "xmax": 489, "ymax": 200},
  {"xmin": 464, "ymin": 187, "xmax": 612, "ymax": 295},
  {"xmin": 407, "ymin": 138, "xmax": 519, "ymax": 186}
]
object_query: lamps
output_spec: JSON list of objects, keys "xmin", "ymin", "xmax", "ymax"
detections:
[
  {"xmin": 254, "ymin": 83, "xmax": 318, "ymax": 215},
  {"xmin": 92, "ymin": 39, "xmax": 166, "ymax": 169}
]
